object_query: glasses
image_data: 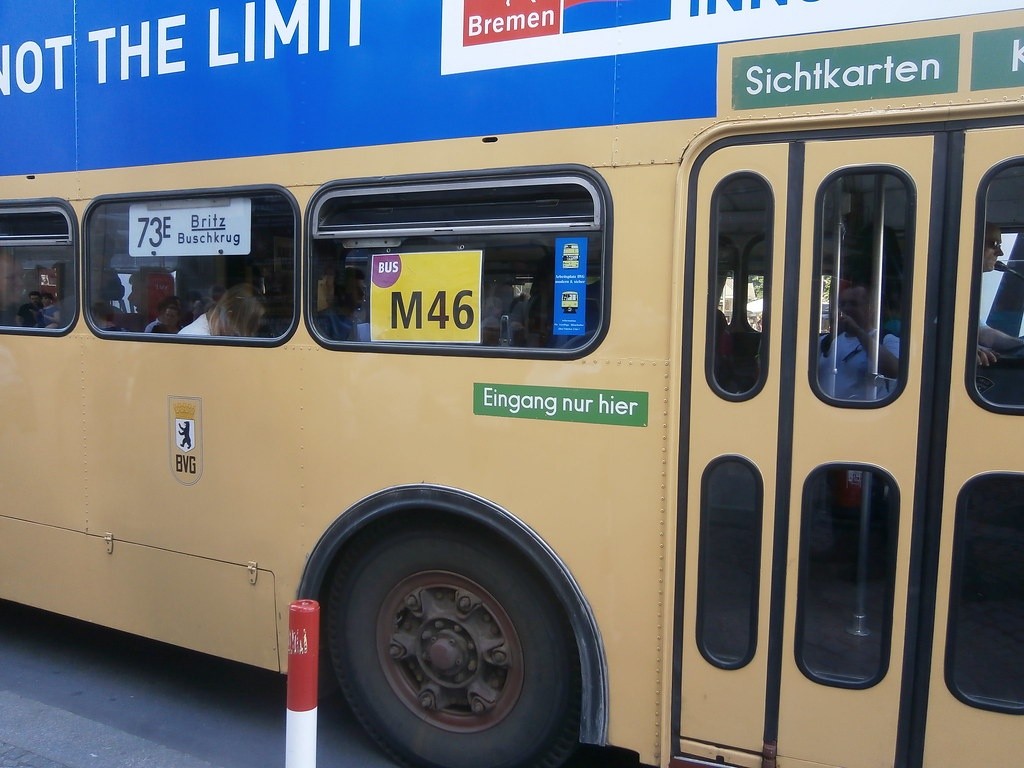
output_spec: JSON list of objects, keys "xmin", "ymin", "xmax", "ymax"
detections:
[{"xmin": 985, "ymin": 239, "xmax": 1002, "ymax": 248}]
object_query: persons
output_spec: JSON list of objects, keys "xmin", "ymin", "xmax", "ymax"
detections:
[
  {"xmin": 129, "ymin": 266, "xmax": 175, "ymax": 313},
  {"xmin": 178, "ymin": 282, "xmax": 266, "ymax": 336},
  {"xmin": 318, "ymin": 267, "xmax": 366, "ymax": 341},
  {"xmin": 976, "ymin": 220, "xmax": 1024, "ymax": 366},
  {"xmin": 15, "ymin": 292, "xmax": 60, "ymax": 329},
  {"xmin": 0, "ymin": 254, "xmax": 25, "ymax": 326},
  {"xmin": 91, "ymin": 300, "xmax": 128, "ymax": 332},
  {"xmin": 144, "ymin": 297, "xmax": 182, "ymax": 333},
  {"xmin": 817, "ymin": 285, "xmax": 899, "ymax": 400}
]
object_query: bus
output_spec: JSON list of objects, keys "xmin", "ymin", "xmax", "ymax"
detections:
[{"xmin": 1, "ymin": 0, "xmax": 1024, "ymax": 766}]
